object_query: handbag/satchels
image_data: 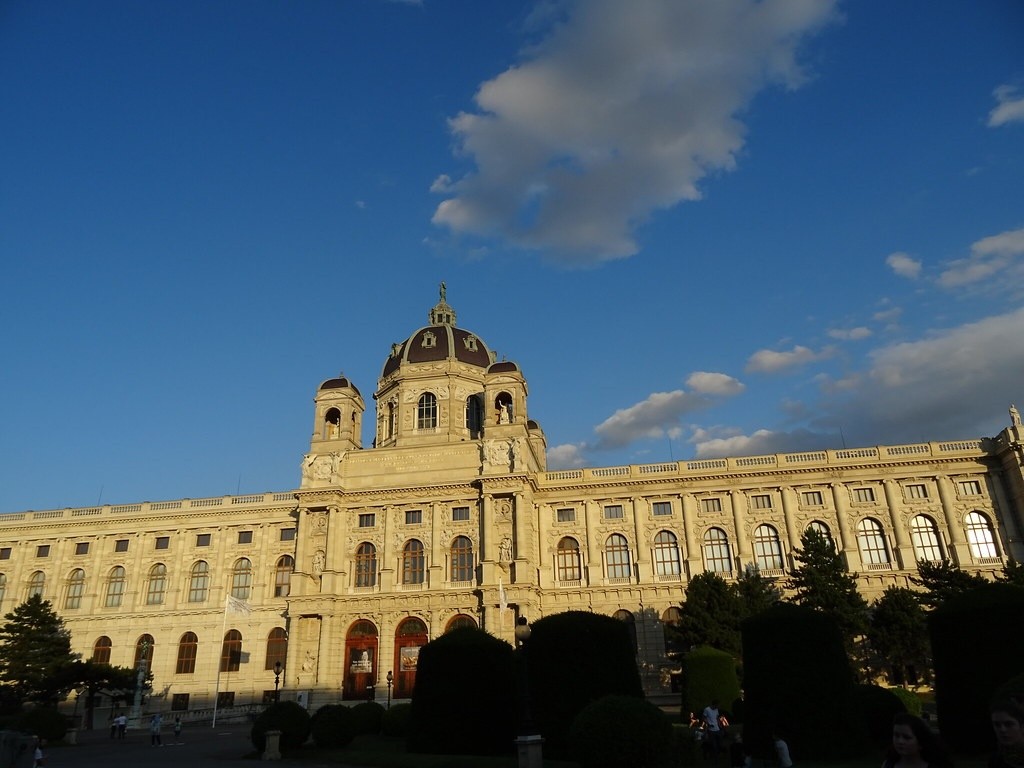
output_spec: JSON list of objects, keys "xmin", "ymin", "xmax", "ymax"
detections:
[{"xmin": 124, "ymin": 727, "xmax": 127, "ymax": 734}]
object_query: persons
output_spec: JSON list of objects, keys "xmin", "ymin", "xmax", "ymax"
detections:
[
  {"xmin": 881, "ymin": 713, "xmax": 953, "ymax": 768},
  {"xmin": 174, "ymin": 718, "xmax": 182, "ymax": 744},
  {"xmin": 499, "ymin": 401, "xmax": 510, "ymax": 425},
  {"xmin": 700, "ymin": 699, "xmax": 724, "ymax": 760},
  {"xmin": 300, "ymin": 454, "xmax": 311, "ymax": 474},
  {"xmin": 500, "ymin": 539, "xmax": 511, "ymax": 561},
  {"xmin": 313, "ymin": 552, "xmax": 325, "ymax": 571},
  {"xmin": 773, "ymin": 731, "xmax": 792, "ymax": 768},
  {"xmin": 481, "ymin": 438, "xmax": 492, "ymax": 460},
  {"xmin": 32, "ymin": 739, "xmax": 48, "ymax": 768},
  {"xmin": 1010, "ymin": 403, "xmax": 1021, "ymax": 425},
  {"xmin": 984, "ymin": 701, "xmax": 1024, "ymax": 768},
  {"xmin": 730, "ymin": 733, "xmax": 750, "ymax": 768},
  {"xmin": 330, "ymin": 450, "xmax": 342, "ymax": 472},
  {"xmin": 151, "ymin": 714, "xmax": 164, "ymax": 747},
  {"xmin": 110, "ymin": 713, "xmax": 127, "ymax": 740},
  {"xmin": 507, "ymin": 437, "xmax": 521, "ymax": 462}
]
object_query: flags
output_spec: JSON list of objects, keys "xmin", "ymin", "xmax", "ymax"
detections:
[
  {"xmin": 225, "ymin": 595, "xmax": 251, "ymax": 615},
  {"xmin": 499, "ymin": 577, "xmax": 507, "ymax": 617}
]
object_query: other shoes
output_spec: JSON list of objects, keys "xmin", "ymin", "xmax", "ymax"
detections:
[
  {"xmin": 158, "ymin": 744, "xmax": 164, "ymax": 747},
  {"xmin": 152, "ymin": 745, "xmax": 156, "ymax": 747}
]
